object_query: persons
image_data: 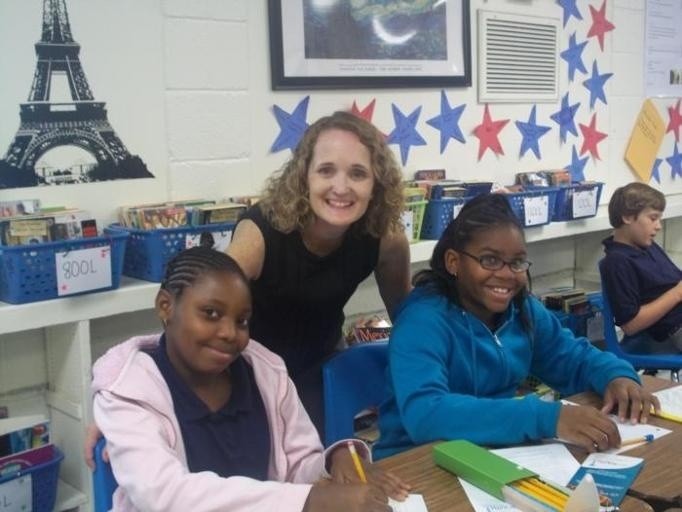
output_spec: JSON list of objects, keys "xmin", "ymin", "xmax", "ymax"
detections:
[
  {"xmin": 84, "ymin": 109, "xmax": 413, "ymax": 473},
  {"xmin": 600, "ymin": 183, "xmax": 681, "ymax": 355},
  {"xmin": 373, "ymin": 193, "xmax": 662, "ymax": 464},
  {"xmin": 93, "ymin": 244, "xmax": 411, "ymax": 512}
]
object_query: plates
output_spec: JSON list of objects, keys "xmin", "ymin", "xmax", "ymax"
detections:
[{"xmin": 0, "ymin": 191, "xmax": 682, "ymax": 511}]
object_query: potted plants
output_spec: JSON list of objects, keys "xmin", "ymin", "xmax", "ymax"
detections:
[{"xmin": 265, "ymin": 0, "xmax": 472, "ymax": 91}]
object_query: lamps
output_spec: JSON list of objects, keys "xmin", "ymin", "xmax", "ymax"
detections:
[
  {"xmin": 92, "ymin": 437, "xmax": 120, "ymax": 510},
  {"xmin": 597, "ymin": 258, "xmax": 681, "ymax": 376},
  {"xmin": 322, "ymin": 339, "xmax": 414, "ymax": 462}
]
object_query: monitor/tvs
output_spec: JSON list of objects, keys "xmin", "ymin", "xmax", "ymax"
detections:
[
  {"xmin": 397, "ymin": 167, "xmax": 573, "ymax": 200},
  {"xmin": 0, "ymin": 408, "xmax": 55, "ymax": 478},
  {"xmin": 1, "ymin": 194, "xmax": 261, "ymax": 248}
]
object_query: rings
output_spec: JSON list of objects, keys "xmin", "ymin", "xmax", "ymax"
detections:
[
  {"xmin": 619, "ymin": 433, "xmax": 654, "ymax": 447},
  {"xmin": 517, "ymin": 479, "xmax": 567, "ymax": 508},
  {"xmin": 508, "ymin": 482, "xmax": 565, "ymax": 512},
  {"xmin": 347, "ymin": 441, "xmax": 367, "ymax": 483},
  {"xmin": 523, "ymin": 477, "xmax": 570, "ymax": 501}
]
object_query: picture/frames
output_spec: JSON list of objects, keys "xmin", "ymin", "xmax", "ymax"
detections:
[
  {"xmin": 546, "ymin": 374, "xmax": 682, "ymax": 512},
  {"xmin": 229, "ymin": 438, "xmax": 657, "ymax": 511}
]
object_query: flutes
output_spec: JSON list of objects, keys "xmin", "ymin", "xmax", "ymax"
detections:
[{"xmin": 431, "ymin": 439, "xmax": 575, "ymax": 512}]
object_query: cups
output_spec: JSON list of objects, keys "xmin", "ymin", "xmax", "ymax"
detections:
[{"xmin": 459, "ymin": 251, "xmax": 532, "ymax": 273}]
